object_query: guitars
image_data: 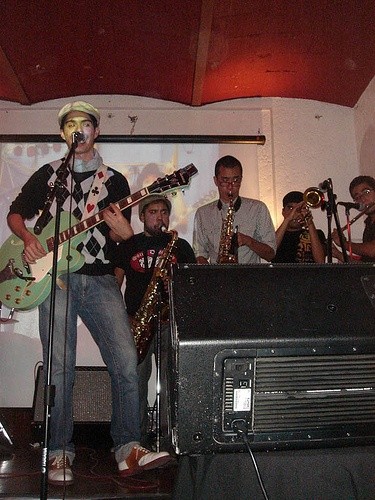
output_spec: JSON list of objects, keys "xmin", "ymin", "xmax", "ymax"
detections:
[
  {"xmin": 0, "ymin": 163, "xmax": 198, "ymax": 311},
  {"xmin": 217, "ymin": 190, "xmax": 238, "ymax": 264}
]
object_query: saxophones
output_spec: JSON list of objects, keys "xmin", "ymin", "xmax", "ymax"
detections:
[{"xmin": 129, "ymin": 226, "xmax": 178, "ymax": 366}]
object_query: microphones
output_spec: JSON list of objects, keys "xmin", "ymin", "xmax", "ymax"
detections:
[
  {"xmin": 72, "ymin": 132, "xmax": 87, "ymax": 143},
  {"xmin": 157, "ymin": 223, "xmax": 166, "ymax": 233},
  {"xmin": 338, "ymin": 201, "xmax": 366, "ymax": 211}
]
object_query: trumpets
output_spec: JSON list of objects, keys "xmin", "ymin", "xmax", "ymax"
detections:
[{"xmin": 297, "ymin": 188, "xmax": 325, "ymax": 223}]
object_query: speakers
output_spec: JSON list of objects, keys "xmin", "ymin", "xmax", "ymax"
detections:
[
  {"xmin": 160, "ymin": 264, "xmax": 375, "ymax": 455},
  {"xmin": 31, "ymin": 364, "xmax": 113, "ymax": 445}
]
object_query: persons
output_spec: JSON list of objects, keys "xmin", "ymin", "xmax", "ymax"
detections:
[
  {"xmin": 332, "ymin": 176, "xmax": 375, "ymax": 263},
  {"xmin": 7, "ymin": 101, "xmax": 172, "ymax": 486},
  {"xmin": 271, "ymin": 191, "xmax": 327, "ymax": 263},
  {"xmin": 193, "ymin": 155, "xmax": 277, "ymax": 264},
  {"xmin": 115, "ymin": 194, "xmax": 198, "ymax": 440}
]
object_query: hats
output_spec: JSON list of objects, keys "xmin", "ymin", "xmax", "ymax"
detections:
[
  {"xmin": 57, "ymin": 101, "xmax": 100, "ymax": 127},
  {"xmin": 139, "ymin": 195, "xmax": 171, "ymax": 221}
]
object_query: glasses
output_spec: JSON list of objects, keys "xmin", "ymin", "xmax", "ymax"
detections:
[
  {"xmin": 353, "ymin": 188, "xmax": 374, "ymax": 202},
  {"xmin": 217, "ymin": 177, "xmax": 241, "ymax": 187}
]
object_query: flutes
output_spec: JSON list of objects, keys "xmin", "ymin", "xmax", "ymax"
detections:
[{"xmin": 326, "ymin": 201, "xmax": 375, "ymax": 243}]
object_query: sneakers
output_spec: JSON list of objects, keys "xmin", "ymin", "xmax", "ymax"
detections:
[
  {"xmin": 117, "ymin": 445, "xmax": 170, "ymax": 477},
  {"xmin": 47, "ymin": 455, "xmax": 73, "ymax": 486}
]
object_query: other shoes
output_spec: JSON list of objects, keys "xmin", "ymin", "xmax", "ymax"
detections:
[
  {"xmin": 140, "ymin": 431, "xmax": 147, "ymax": 443},
  {"xmin": 160, "ymin": 432, "xmax": 171, "ymax": 444}
]
